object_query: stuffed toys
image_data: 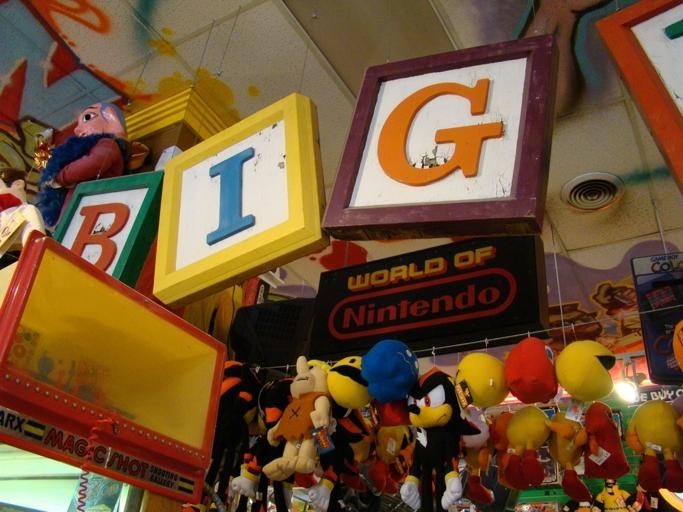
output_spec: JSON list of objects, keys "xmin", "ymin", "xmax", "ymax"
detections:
[
  {"xmin": 204, "ymin": 317, "xmax": 683, "ymax": 512},
  {"xmin": 33, "ymin": 101, "xmax": 128, "ymax": 232},
  {"xmin": 0, "ymin": 167, "xmax": 47, "ymax": 262}
]
